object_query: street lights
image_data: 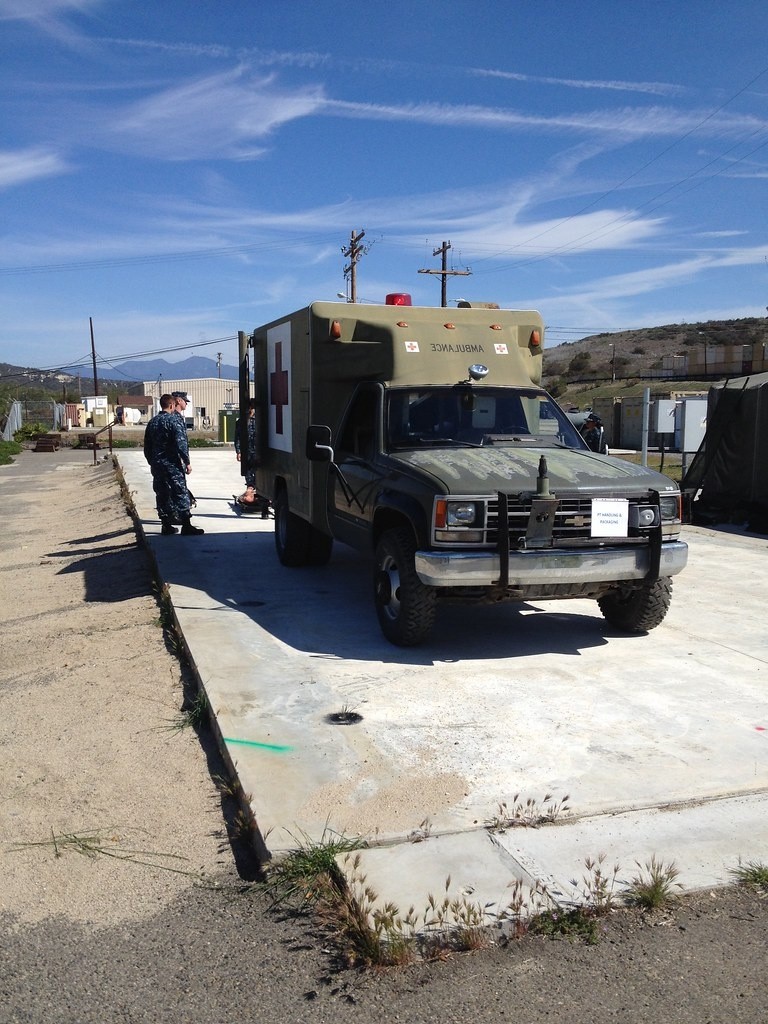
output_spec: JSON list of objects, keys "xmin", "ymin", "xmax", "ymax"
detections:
[
  {"xmin": 217, "ymin": 352, "xmax": 222, "ymax": 378},
  {"xmin": 609, "ymin": 343, "xmax": 616, "ymax": 383},
  {"xmin": 699, "ymin": 332, "xmax": 708, "ymax": 381}
]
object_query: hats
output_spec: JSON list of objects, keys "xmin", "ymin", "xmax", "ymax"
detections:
[
  {"xmin": 584, "ymin": 414, "xmax": 601, "ymax": 424},
  {"xmin": 172, "ymin": 392, "xmax": 190, "ymax": 402}
]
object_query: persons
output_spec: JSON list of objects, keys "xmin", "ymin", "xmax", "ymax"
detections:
[
  {"xmin": 173, "ymin": 391, "xmax": 191, "ymax": 475},
  {"xmin": 583, "ymin": 414, "xmax": 606, "ymax": 453},
  {"xmin": 235, "ymin": 399, "xmax": 255, "ymax": 490},
  {"xmin": 144, "ymin": 394, "xmax": 204, "ymax": 535}
]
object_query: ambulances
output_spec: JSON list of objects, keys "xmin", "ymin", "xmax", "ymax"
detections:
[{"xmin": 238, "ymin": 293, "xmax": 691, "ymax": 648}]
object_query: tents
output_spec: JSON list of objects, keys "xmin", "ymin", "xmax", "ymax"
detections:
[{"xmin": 701, "ymin": 372, "xmax": 768, "ymax": 524}]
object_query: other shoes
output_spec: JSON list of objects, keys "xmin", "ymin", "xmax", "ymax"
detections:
[{"xmin": 162, "ymin": 519, "xmax": 204, "ymax": 535}]
